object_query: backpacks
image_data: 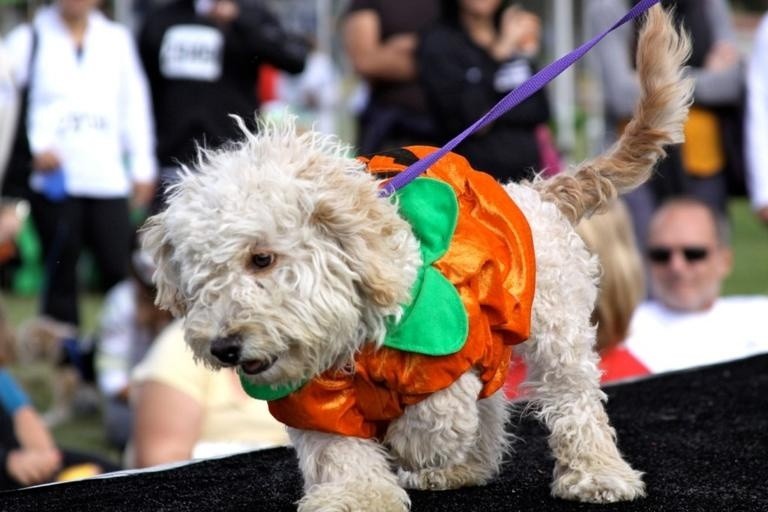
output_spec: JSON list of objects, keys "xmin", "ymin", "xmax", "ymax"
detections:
[{"xmin": 0, "ymin": 22, "xmax": 38, "ymax": 198}]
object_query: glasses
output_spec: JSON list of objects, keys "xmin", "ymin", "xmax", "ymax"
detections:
[{"xmin": 647, "ymin": 245, "xmax": 707, "ymax": 261}]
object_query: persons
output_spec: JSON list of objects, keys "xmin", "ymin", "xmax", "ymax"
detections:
[
  {"xmin": 340, "ymin": 0, "xmax": 561, "ymax": 186},
  {"xmin": 580, "ymin": 0, "xmax": 768, "ymax": 294},
  {"xmin": 0, "ymin": 0, "xmax": 336, "ymax": 490},
  {"xmin": 505, "ymin": 195, "xmax": 768, "ymax": 400}
]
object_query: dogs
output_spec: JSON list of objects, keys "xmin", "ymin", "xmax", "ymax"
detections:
[{"xmin": 134, "ymin": 1, "xmax": 700, "ymax": 512}]
